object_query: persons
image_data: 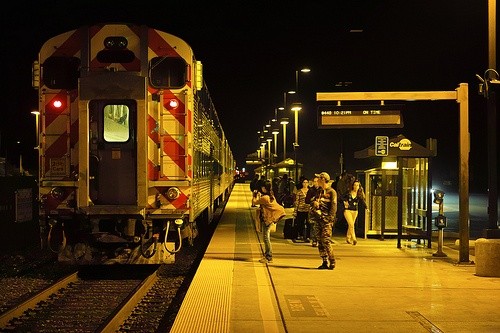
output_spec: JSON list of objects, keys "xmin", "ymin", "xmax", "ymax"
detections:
[
  {"xmin": 343, "ymin": 181, "xmax": 369, "ymax": 245},
  {"xmin": 315, "ymin": 172, "xmax": 337, "ymax": 268},
  {"xmin": 291, "ymin": 179, "xmax": 310, "ymax": 242},
  {"xmin": 252, "ymin": 182, "xmax": 286, "ymax": 263},
  {"xmin": 250, "ymin": 173, "xmax": 304, "ymax": 207},
  {"xmin": 305, "ymin": 177, "xmax": 322, "ymax": 246}
]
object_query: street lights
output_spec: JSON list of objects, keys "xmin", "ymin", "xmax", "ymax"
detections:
[
  {"xmin": 291, "ymin": 68, "xmax": 311, "ymax": 182},
  {"xmin": 256, "ymin": 106, "xmax": 284, "ymax": 182},
  {"xmin": 280, "ymin": 90, "xmax": 295, "ymax": 161},
  {"xmin": 31, "ymin": 111, "xmax": 40, "ymax": 146}
]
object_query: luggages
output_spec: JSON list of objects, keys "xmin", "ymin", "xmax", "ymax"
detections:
[{"xmin": 283, "ymin": 215, "xmax": 298, "ymax": 239}]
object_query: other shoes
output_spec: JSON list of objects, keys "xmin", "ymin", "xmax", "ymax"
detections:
[
  {"xmin": 252, "ymin": 204, "xmax": 257, "ymax": 207},
  {"xmin": 312, "ymin": 242, "xmax": 317, "ymax": 247},
  {"xmin": 329, "ymin": 259, "xmax": 335, "ymax": 268},
  {"xmin": 259, "ymin": 258, "xmax": 272, "ymax": 263},
  {"xmin": 306, "ymin": 238, "xmax": 310, "ymax": 243},
  {"xmin": 347, "ymin": 240, "xmax": 352, "ymax": 244},
  {"xmin": 353, "ymin": 240, "xmax": 356, "ymax": 244},
  {"xmin": 318, "ymin": 262, "xmax": 329, "ymax": 269}
]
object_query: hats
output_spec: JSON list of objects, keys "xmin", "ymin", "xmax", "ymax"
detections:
[{"xmin": 315, "ymin": 172, "xmax": 330, "ymax": 181}]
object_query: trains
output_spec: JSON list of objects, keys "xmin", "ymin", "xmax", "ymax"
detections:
[{"xmin": 32, "ymin": 22, "xmax": 237, "ymax": 267}]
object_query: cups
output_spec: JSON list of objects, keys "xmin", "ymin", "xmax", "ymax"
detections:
[{"xmin": 345, "ymin": 200, "xmax": 348, "ymax": 208}]
